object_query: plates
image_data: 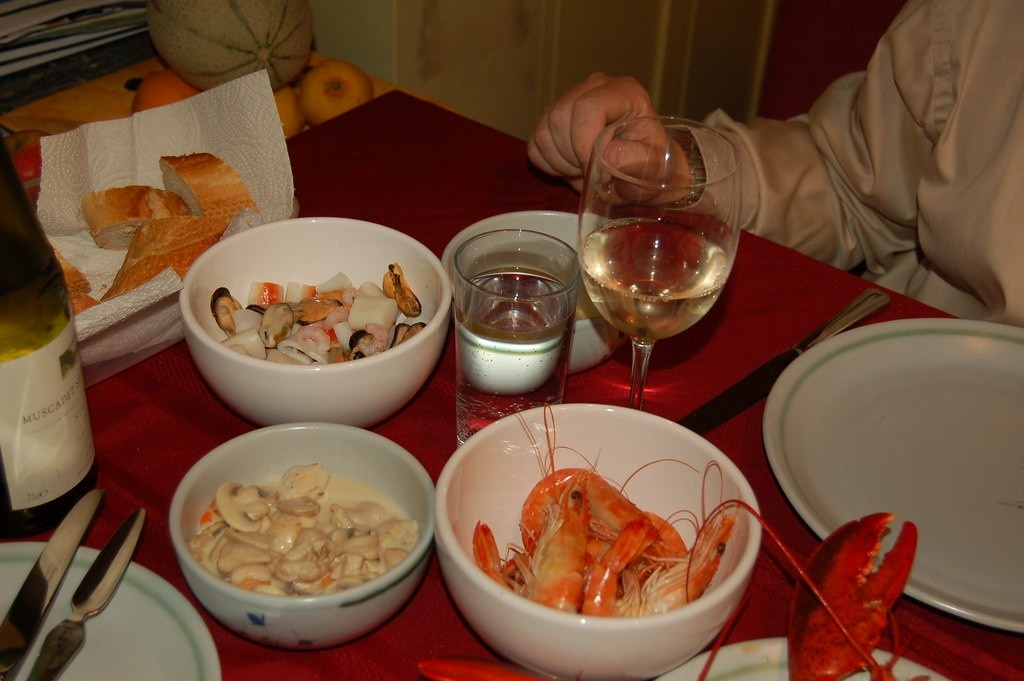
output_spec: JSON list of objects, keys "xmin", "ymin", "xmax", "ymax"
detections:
[
  {"xmin": 650, "ymin": 637, "xmax": 952, "ymax": 681},
  {"xmin": 0, "ymin": 542, "xmax": 223, "ymax": 681},
  {"xmin": 763, "ymin": 318, "xmax": 1024, "ymax": 634}
]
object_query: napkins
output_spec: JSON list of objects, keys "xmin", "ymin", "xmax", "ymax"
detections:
[{"xmin": 35, "ymin": 68, "xmax": 295, "ymax": 368}]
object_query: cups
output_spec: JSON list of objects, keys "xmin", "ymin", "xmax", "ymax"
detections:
[{"xmin": 452, "ymin": 231, "xmax": 581, "ymax": 450}]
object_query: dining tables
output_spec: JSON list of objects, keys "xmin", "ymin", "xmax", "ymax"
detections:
[{"xmin": 0, "ymin": 46, "xmax": 1024, "ymax": 681}]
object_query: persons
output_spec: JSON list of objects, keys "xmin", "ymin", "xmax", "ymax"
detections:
[{"xmin": 526, "ymin": 0, "xmax": 1024, "ymax": 328}]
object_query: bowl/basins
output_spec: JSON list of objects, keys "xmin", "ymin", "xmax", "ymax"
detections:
[
  {"xmin": 179, "ymin": 217, "xmax": 452, "ymax": 429},
  {"xmin": 170, "ymin": 422, "xmax": 435, "ymax": 648},
  {"xmin": 441, "ymin": 210, "xmax": 632, "ymax": 376},
  {"xmin": 434, "ymin": 403, "xmax": 763, "ymax": 681}
]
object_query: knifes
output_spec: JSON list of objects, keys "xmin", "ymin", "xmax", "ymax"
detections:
[
  {"xmin": 1, "ymin": 487, "xmax": 105, "ymax": 680},
  {"xmin": 673, "ymin": 286, "xmax": 892, "ymax": 438}
]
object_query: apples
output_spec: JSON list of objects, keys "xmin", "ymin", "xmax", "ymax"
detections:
[
  {"xmin": 2, "ymin": 128, "xmax": 57, "ymax": 210},
  {"xmin": 132, "ymin": 58, "xmax": 376, "ymax": 137}
]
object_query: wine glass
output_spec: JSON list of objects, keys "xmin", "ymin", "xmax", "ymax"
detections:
[{"xmin": 580, "ymin": 114, "xmax": 744, "ymax": 410}]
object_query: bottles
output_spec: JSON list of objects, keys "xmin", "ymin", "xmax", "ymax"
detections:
[
  {"xmin": 0, "ymin": 138, "xmax": 99, "ymax": 539},
  {"xmin": 28, "ymin": 507, "xmax": 149, "ymax": 681}
]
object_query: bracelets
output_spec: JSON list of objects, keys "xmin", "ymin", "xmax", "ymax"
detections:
[{"xmin": 669, "ymin": 134, "xmax": 706, "ymax": 208}]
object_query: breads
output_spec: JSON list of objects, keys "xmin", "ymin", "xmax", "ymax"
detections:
[
  {"xmin": 160, "ymin": 155, "xmax": 259, "ymax": 225},
  {"xmin": 80, "ymin": 185, "xmax": 197, "ymax": 249},
  {"xmin": 51, "ymin": 214, "xmax": 228, "ymax": 316}
]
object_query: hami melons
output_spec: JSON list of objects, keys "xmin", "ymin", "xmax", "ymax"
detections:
[{"xmin": 148, "ymin": 0, "xmax": 315, "ymax": 94}]
